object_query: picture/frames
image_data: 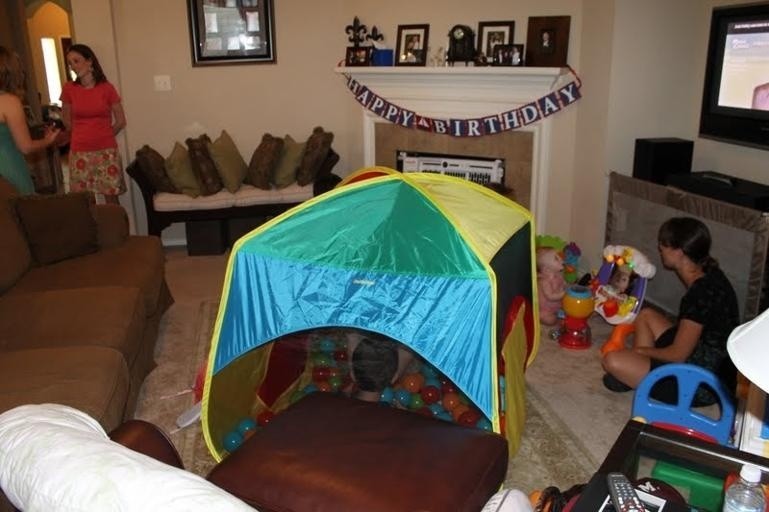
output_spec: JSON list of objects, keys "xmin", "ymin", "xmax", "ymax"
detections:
[
  {"xmin": 345, "ymin": 46, "xmax": 370, "ymax": 67},
  {"xmin": 393, "ymin": 23, "xmax": 429, "ymax": 66},
  {"xmin": 524, "ymin": 16, "xmax": 572, "ymax": 67},
  {"xmin": 492, "ymin": 44, "xmax": 524, "ymax": 67},
  {"xmin": 475, "ymin": 20, "xmax": 515, "ymax": 65},
  {"xmin": 186, "ymin": 0, "xmax": 277, "ymax": 69}
]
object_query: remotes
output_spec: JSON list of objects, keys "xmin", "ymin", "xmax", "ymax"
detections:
[
  {"xmin": 606, "ymin": 471, "xmax": 645, "ymax": 512},
  {"xmin": 701, "ymin": 172, "xmax": 734, "ymax": 188}
]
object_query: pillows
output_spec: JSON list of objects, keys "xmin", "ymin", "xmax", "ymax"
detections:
[
  {"xmin": 13, "ymin": 190, "xmax": 101, "ymax": 265},
  {"xmin": 134, "ymin": 125, "xmax": 334, "ymax": 199},
  {"xmin": 0, "ymin": 400, "xmax": 258, "ymax": 512}
]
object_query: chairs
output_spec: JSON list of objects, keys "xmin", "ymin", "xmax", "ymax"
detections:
[{"xmin": 0, "ymin": 391, "xmax": 534, "ymax": 512}]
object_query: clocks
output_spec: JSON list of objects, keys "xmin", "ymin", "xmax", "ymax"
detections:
[{"xmin": 445, "ymin": 24, "xmax": 474, "ymax": 67}]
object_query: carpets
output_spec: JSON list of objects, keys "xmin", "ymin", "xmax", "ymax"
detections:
[{"xmin": 179, "ymin": 296, "xmax": 602, "ymax": 500}]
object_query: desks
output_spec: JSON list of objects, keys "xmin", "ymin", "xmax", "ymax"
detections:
[{"xmin": 567, "ymin": 417, "xmax": 769, "ymax": 512}]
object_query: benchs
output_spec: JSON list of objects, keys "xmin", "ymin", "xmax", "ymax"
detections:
[{"xmin": 125, "ymin": 146, "xmax": 341, "ymax": 239}]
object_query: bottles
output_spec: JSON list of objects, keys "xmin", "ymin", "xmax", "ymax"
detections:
[{"xmin": 723, "ymin": 464, "xmax": 766, "ymax": 512}]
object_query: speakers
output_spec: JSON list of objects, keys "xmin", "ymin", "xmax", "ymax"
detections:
[{"xmin": 633, "ymin": 137, "xmax": 694, "ymax": 185}]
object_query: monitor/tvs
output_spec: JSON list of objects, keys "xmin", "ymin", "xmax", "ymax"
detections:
[{"xmin": 697, "ymin": 4, "xmax": 769, "ymax": 150}]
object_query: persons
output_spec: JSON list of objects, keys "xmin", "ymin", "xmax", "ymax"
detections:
[
  {"xmin": 512, "ymin": 47, "xmax": 521, "ymax": 66},
  {"xmin": 491, "ymin": 34, "xmax": 502, "ymax": 58},
  {"xmin": 600, "ymin": 213, "xmax": 746, "ymax": 408},
  {"xmin": 405, "ymin": 35, "xmax": 419, "ymax": 49},
  {"xmin": 536, "ymin": 244, "xmax": 567, "ymax": 327},
  {"xmin": 539, "ymin": 31, "xmax": 552, "ymax": 54},
  {"xmin": 59, "ymin": 43, "xmax": 129, "ymax": 204},
  {"xmin": 495, "ymin": 48, "xmax": 506, "ymax": 64},
  {"xmin": 0, "ymin": 45, "xmax": 63, "ymax": 197}
]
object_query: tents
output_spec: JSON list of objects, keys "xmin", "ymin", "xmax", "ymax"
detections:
[{"xmin": 200, "ymin": 160, "xmax": 542, "ymax": 464}]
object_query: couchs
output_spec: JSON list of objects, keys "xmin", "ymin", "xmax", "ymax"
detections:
[{"xmin": 0, "ymin": 198, "xmax": 166, "ymax": 511}]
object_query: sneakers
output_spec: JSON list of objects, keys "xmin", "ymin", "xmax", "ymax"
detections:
[{"xmin": 603, "ymin": 373, "xmax": 633, "ymax": 392}]
